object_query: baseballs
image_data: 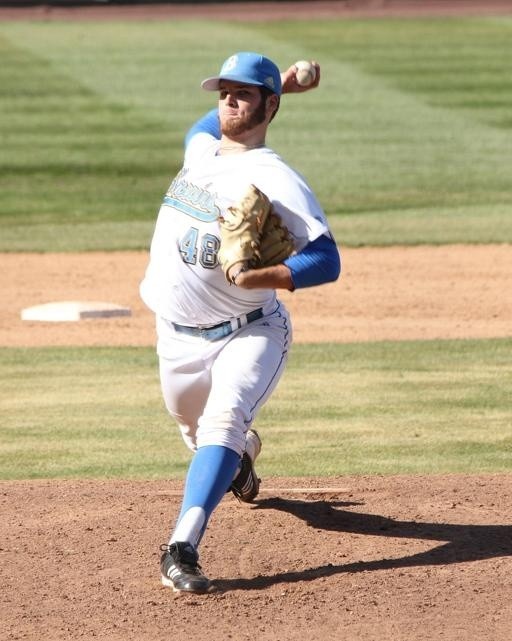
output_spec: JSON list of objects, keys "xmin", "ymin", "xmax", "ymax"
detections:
[{"xmin": 294, "ymin": 59, "xmax": 316, "ymax": 87}]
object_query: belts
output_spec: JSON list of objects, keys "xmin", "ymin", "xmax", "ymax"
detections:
[{"xmin": 172, "ymin": 308, "xmax": 264, "ymax": 340}]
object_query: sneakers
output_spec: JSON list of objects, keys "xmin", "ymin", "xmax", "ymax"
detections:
[
  {"xmin": 231, "ymin": 429, "xmax": 261, "ymax": 502},
  {"xmin": 160, "ymin": 542, "xmax": 210, "ymax": 592}
]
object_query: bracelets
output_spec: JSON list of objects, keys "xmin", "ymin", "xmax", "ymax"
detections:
[{"xmin": 228, "ymin": 266, "xmax": 248, "ymax": 286}]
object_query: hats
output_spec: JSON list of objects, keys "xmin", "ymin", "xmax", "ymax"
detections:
[{"xmin": 201, "ymin": 51, "xmax": 282, "ymax": 97}]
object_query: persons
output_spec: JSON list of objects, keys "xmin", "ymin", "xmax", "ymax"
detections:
[{"xmin": 138, "ymin": 48, "xmax": 342, "ymax": 595}]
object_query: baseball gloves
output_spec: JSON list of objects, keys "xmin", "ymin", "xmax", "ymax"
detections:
[{"xmin": 216, "ymin": 183, "xmax": 293, "ymax": 286}]
